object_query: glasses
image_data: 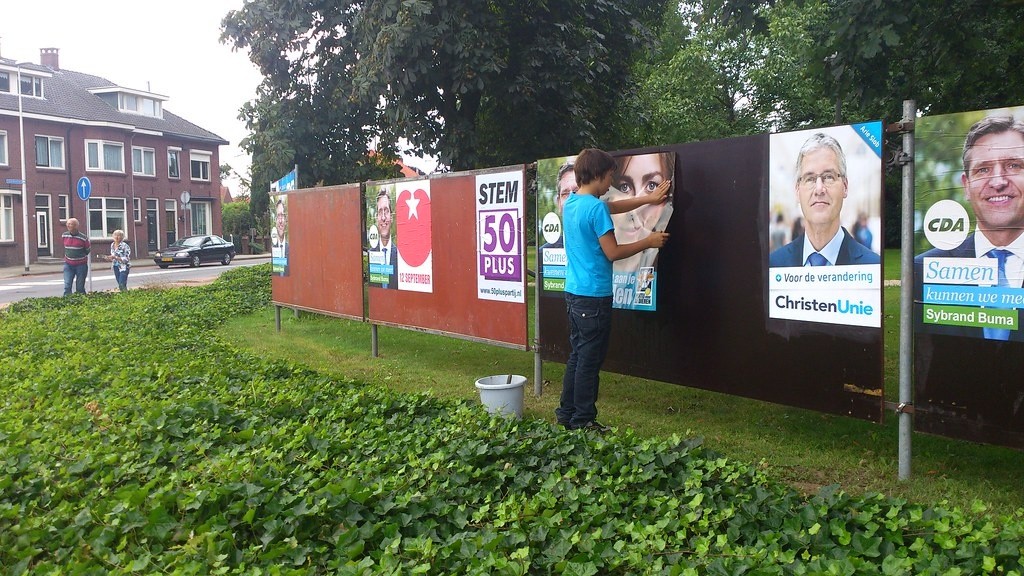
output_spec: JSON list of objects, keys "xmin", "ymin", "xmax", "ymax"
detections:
[{"xmin": 802, "ymin": 170, "xmax": 841, "ymax": 190}]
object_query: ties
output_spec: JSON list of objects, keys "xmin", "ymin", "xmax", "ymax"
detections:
[
  {"xmin": 280, "ymin": 245, "xmax": 283, "ymax": 276},
  {"xmin": 382, "ymin": 248, "xmax": 388, "ymax": 288},
  {"xmin": 986, "ymin": 249, "xmax": 1014, "ymax": 340},
  {"xmin": 808, "ymin": 252, "xmax": 827, "ymax": 266}
]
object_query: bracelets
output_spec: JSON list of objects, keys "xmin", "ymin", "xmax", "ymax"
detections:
[{"xmin": 116, "ymin": 256, "xmax": 117, "ymax": 258}]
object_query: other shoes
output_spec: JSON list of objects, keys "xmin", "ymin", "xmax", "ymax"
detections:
[
  {"xmin": 557, "ymin": 418, "xmax": 571, "ymax": 430},
  {"xmin": 568, "ymin": 417, "xmax": 616, "ymax": 436}
]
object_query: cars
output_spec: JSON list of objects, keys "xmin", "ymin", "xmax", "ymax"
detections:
[{"xmin": 154, "ymin": 234, "xmax": 237, "ymax": 270}]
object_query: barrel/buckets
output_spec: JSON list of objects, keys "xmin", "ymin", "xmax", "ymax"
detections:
[{"xmin": 475, "ymin": 375, "xmax": 527, "ymax": 424}]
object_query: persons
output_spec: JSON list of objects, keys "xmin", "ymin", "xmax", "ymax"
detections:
[
  {"xmin": 606, "ymin": 154, "xmax": 673, "ymax": 272},
  {"xmin": 538, "ymin": 164, "xmax": 580, "ymax": 264},
  {"xmin": 915, "ymin": 117, "xmax": 1024, "ymax": 344},
  {"xmin": 61, "ymin": 217, "xmax": 90, "ymax": 296},
  {"xmin": 554, "ymin": 148, "xmax": 672, "ymax": 433},
  {"xmin": 273, "ymin": 199, "xmax": 289, "ymax": 276},
  {"xmin": 369, "ymin": 188, "xmax": 398, "ymax": 289},
  {"xmin": 769, "ymin": 132, "xmax": 880, "ymax": 267},
  {"xmin": 107, "ymin": 230, "xmax": 132, "ymax": 291}
]
